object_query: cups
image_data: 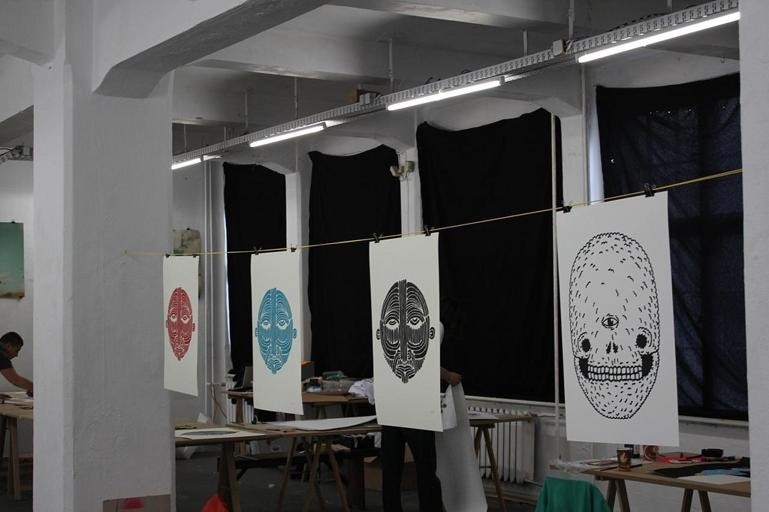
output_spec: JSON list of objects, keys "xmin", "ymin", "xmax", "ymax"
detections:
[{"xmin": 617, "ymin": 448, "xmax": 632, "ymax": 469}]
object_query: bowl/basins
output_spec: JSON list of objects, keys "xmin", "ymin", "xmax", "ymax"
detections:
[{"xmin": 701, "ymin": 449, "xmax": 723, "ymax": 460}]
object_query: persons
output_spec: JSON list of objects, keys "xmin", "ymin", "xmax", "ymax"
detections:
[
  {"xmin": 380, "ymin": 320, "xmax": 462, "ymax": 512},
  {"xmin": 0, "ymin": 332, "xmax": 34, "ymax": 471}
]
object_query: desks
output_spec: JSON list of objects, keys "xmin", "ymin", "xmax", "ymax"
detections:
[
  {"xmin": 548, "ymin": 452, "xmax": 752, "ymax": 512},
  {"xmin": 175, "ymin": 424, "xmax": 264, "ymax": 511},
  {"xmin": 0, "ymin": 397, "xmax": 35, "ymax": 501},
  {"xmin": 230, "ymin": 413, "xmax": 531, "ymax": 508},
  {"xmin": 225, "ymin": 388, "xmax": 376, "ymax": 478}
]
